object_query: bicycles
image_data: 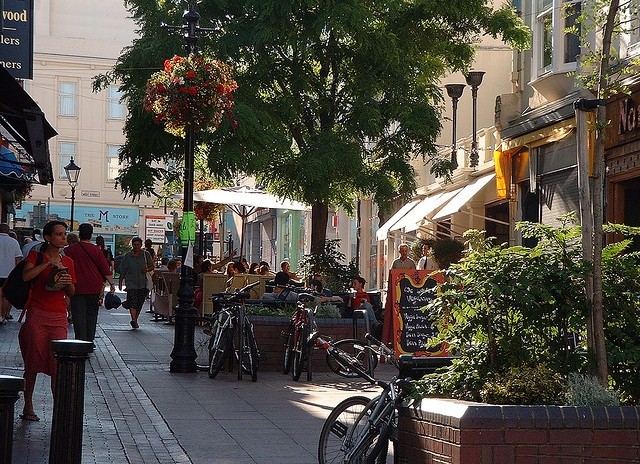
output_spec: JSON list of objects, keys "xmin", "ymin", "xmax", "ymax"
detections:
[
  {"xmin": 277, "ymin": 284, "xmax": 325, "ymax": 381},
  {"xmin": 306, "ymin": 305, "xmax": 378, "ymax": 378},
  {"xmin": 229, "ymin": 281, "xmax": 261, "ymax": 381},
  {"xmin": 195, "ymin": 286, "xmax": 236, "ymax": 378},
  {"xmin": 318, "ymin": 332, "xmax": 414, "ymax": 464}
]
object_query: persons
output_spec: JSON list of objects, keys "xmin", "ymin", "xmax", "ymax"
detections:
[
  {"xmin": 251, "ymin": 263, "xmax": 260, "ymax": 269},
  {"xmin": 195, "ymin": 260, "xmax": 224, "ymax": 289},
  {"xmin": 351, "ymin": 277, "xmax": 385, "ymax": 338},
  {"xmin": 0, "ymin": 223, "xmax": 25, "ymax": 325},
  {"xmin": 226, "ymin": 263, "xmax": 234, "ymax": 277},
  {"xmin": 259, "ymin": 261, "xmax": 269, "ymax": 267},
  {"xmin": 391, "ymin": 244, "xmax": 416, "ymax": 269},
  {"xmin": 21, "ymin": 237, "xmax": 33, "ymax": 249},
  {"xmin": 193, "ymin": 248, "xmax": 238, "ymax": 274},
  {"xmin": 259, "ymin": 271, "xmax": 344, "ymax": 306},
  {"xmin": 167, "ymin": 260, "xmax": 177, "ymax": 273},
  {"xmin": 64, "ymin": 223, "xmax": 115, "ymax": 350},
  {"xmin": 416, "ymin": 243, "xmax": 443, "ymax": 270},
  {"xmin": 233, "ymin": 256, "xmax": 249, "ymax": 274},
  {"xmin": 32, "ymin": 229, "xmax": 43, "ymax": 241},
  {"xmin": 141, "ymin": 239, "xmax": 156, "ymax": 299},
  {"xmin": 7, "ymin": 232, "xmax": 18, "ymax": 240},
  {"xmin": 314, "ymin": 275, "xmax": 323, "ymax": 285},
  {"xmin": 119, "ymin": 237, "xmax": 155, "ymax": 328},
  {"xmin": 157, "ymin": 244, "xmax": 172, "ymax": 260},
  {"xmin": 260, "ymin": 265, "xmax": 276, "ymax": 282},
  {"xmin": 18, "ymin": 220, "xmax": 79, "ymax": 421},
  {"xmin": 309, "ymin": 279, "xmax": 332, "ymax": 297},
  {"xmin": 96, "ymin": 236, "xmax": 117, "ymax": 306},
  {"xmin": 154, "ymin": 257, "xmax": 169, "ymax": 273},
  {"xmin": 59, "ymin": 233, "xmax": 80, "ymax": 324},
  {"xmin": 248, "ymin": 268, "xmax": 260, "ymax": 275},
  {"xmin": 174, "ymin": 258, "xmax": 183, "ymax": 274},
  {"xmin": 280, "ymin": 261, "xmax": 302, "ymax": 282}
]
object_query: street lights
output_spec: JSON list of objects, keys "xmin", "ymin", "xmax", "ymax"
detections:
[{"xmin": 64, "ymin": 156, "xmax": 81, "ymax": 232}]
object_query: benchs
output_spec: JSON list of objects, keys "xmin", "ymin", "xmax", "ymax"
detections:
[
  {"xmin": 331, "ymin": 292, "xmax": 381, "ymax": 319},
  {"xmin": 237, "ymin": 299, "xmax": 342, "ymax": 308}
]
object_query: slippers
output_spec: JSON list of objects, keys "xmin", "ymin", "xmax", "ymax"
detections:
[
  {"xmin": 19, "ymin": 413, "xmax": 40, "ymax": 421},
  {"xmin": 4, "ymin": 314, "xmax": 14, "ymax": 319}
]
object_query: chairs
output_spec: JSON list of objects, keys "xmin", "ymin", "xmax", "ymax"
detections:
[
  {"xmin": 161, "ymin": 272, "xmax": 180, "ymax": 309},
  {"xmin": 229, "ymin": 275, "xmax": 269, "ymax": 299},
  {"xmin": 199, "ymin": 273, "xmax": 228, "ymax": 326}
]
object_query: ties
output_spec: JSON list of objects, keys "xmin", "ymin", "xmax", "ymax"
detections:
[{"xmin": 424, "ymin": 258, "xmax": 427, "ymax": 270}]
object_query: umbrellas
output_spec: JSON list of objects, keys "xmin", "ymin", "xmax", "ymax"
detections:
[{"xmin": 166, "ymin": 185, "xmax": 312, "ymax": 264}]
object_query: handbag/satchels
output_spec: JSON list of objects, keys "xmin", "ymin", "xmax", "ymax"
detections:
[
  {"xmin": 146, "ymin": 269, "xmax": 154, "ymax": 290},
  {"xmin": 106, "ymin": 255, "xmax": 112, "ymax": 268},
  {"xmin": 3, "ymin": 258, "xmax": 30, "ymax": 309}
]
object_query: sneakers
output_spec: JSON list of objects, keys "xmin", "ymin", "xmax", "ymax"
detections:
[
  {"xmin": 372, "ymin": 321, "xmax": 382, "ymax": 330},
  {"xmin": 0, "ymin": 315, "xmax": 7, "ymax": 324}
]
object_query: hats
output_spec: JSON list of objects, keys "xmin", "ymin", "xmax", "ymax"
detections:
[
  {"xmin": 35, "ymin": 229, "xmax": 45, "ymax": 242},
  {"xmin": 105, "ymin": 292, "xmax": 121, "ymax": 310}
]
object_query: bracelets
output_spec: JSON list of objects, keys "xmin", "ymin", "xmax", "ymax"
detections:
[
  {"xmin": 227, "ymin": 257, "xmax": 231, "ymax": 262},
  {"xmin": 110, "ymin": 282, "xmax": 116, "ymax": 285}
]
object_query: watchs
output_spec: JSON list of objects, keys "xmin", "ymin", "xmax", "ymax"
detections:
[{"xmin": 330, "ymin": 297, "xmax": 332, "ymax": 301}]
